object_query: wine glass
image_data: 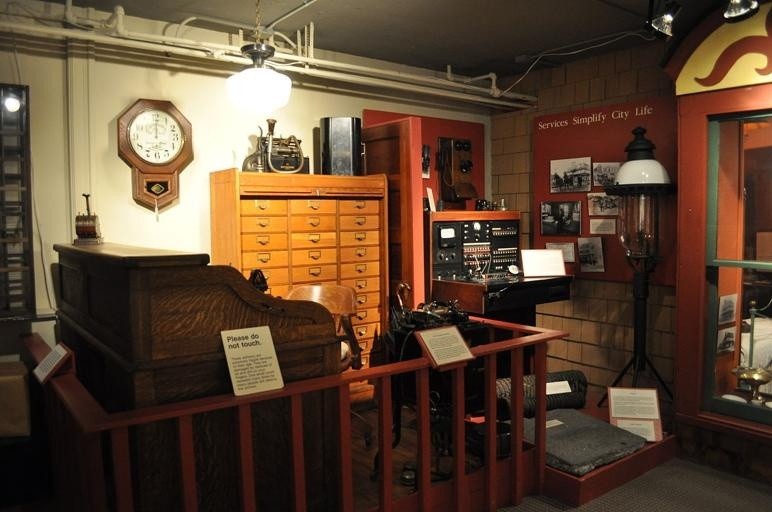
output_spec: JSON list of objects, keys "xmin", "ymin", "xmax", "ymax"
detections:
[{"xmin": 732, "ymin": 367, "xmax": 772, "ymax": 402}]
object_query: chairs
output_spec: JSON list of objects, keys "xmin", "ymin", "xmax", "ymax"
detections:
[{"xmin": 283, "ymin": 284, "xmax": 375, "ymax": 434}]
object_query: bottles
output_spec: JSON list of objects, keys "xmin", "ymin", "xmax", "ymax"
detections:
[
  {"xmin": 437, "ymin": 200, "xmax": 444, "ymax": 212},
  {"xmin": 477, "ymin": 198, "xmax": 508, "ymax": 213}
]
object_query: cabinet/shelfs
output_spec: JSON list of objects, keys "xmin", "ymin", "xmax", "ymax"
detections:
[{"xmin": 209, "ymin": 170, "xmax": 390, "ymax": 401}]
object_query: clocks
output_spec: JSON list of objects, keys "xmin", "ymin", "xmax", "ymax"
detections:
[{"xmin": 117, "ymin": 98, "xmax": 194, "ymax": 210}]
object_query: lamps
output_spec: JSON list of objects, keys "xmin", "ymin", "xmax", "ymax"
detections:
[
  {"xmin": 596, "ymin": 125, "xmax": 676, "ymax": 410},
  {"xmin": 723, "ymin": 0, "xmax": 760, "ymax": 23},
  {"xmin": 222, "ymin": 0, "xmax": 292, "ymax": 121},
  {"xmin": 647, "ymin": 13, "xmax": 675, "ymax": 37},
  {"xmin": 2, "ymin": 86, "xmax": 22, "ymax": 113}
]
